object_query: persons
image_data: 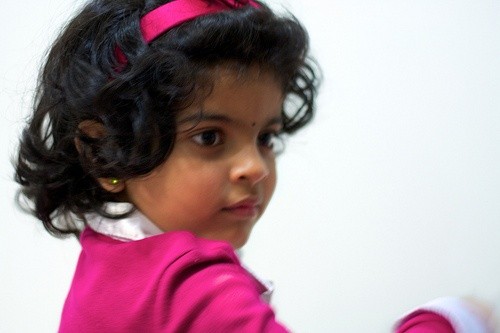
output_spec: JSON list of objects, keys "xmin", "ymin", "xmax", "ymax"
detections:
[{"xmin": 11, "ymin": 0, "xmax": 460, "ymax": 333}]
